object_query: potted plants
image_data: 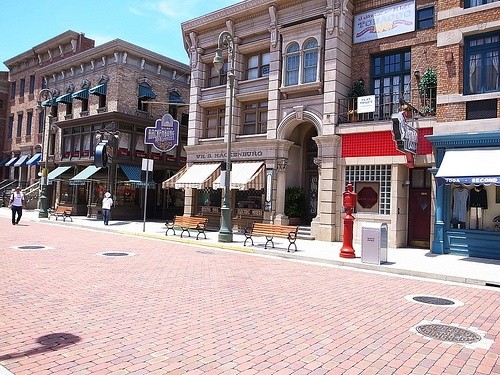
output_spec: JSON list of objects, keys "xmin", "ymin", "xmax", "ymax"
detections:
[{"xmin": 345, "ymin": 79, "xmax": 366, "ymax": 121}]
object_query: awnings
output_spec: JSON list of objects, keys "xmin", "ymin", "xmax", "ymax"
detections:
[
  {"xmin": 39, "ymin": 166, "xmax": 72, "ymax": 184},
  {"xmin": 69, "ymin": 165, "xmax": 102, "ymax": 185},
  {"xmin": 139, "ymin": 85, "xmax": 156, "ymax": 99},
  {"xmin": 26, "ymin": 154, "xmax": 41, "ymax": 165},
  {"xmin": 213, "ymin": 161, "xmax": 266, "ymax": 190},
  {"xmin": 435, "ymin": 147, "xmax": 500, "ymax": 186},
  {"xmin": 14, "ymin": 155, "xmax": 29, "ymax": 166},
  {"xmin": 5, "ymin": 157, "xmax": 19, "ymax": 166},
  {"xmin": 120, "ymin": 164, "xmax": 153, "ymax": 182},
  {"xmin": 0, "ymin": 157, "xmax": 10, "ymax": 166},
  {"xmin": 170, "ymin": 93, "xmax": 185, "ymax": 103},
  {"xmin": 89, "ymin": 84, "xmax": 106, "ymax": 95},
  {"xmin": 43, "ymin": 97, "xmax": 57, "ymax": 107},
  {"xmin": 162, "ymin": 167, "xmax": 186, "ymax": 189},
  {"xmin": 56, "ymin": 93, "xmax": 72, "ymax": 104},
  {"xmin": 73, "ymin": 89, "xmax": 88, "ymax": 100},
  {"xmin": 175, "ymin": 162, "xmax": 222, "ymax": 189}
]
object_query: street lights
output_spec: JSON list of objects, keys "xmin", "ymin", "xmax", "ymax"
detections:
[
  {"xmin": 213, "ymin": 30, "xmax": 234, "ymax": 243},
  {"xmin": 35, "ymin": 89, "xmax": 54, "ymax": 218}
]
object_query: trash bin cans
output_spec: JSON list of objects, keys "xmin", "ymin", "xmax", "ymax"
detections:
[{"xmin": 362, "ymin": 221, "xmax": 387, "ymax": 264}]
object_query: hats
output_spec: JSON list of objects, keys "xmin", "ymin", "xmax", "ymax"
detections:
[{"xmin": 105, "ymin": 193, "xmax": 111, "ymax": 197}]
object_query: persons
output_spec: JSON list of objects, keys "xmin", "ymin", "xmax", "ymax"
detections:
[
  {"xmin": 102, "ymin": 192, "xmax": 113, "ymax": 225},
  {"xmin": 10, "ymin": 187, "xmax": 24, "ymax": 225}
]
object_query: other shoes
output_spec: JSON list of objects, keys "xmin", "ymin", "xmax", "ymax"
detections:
[
  {"xmin": 12, "ymin": 223, "xmax": 16, "ymax": 225},
  {"xmin": 15, "ymin": 222, "xmax": 18, "ymax": 224}
]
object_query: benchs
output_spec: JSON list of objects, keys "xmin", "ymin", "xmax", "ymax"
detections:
[
  {"xmin": 165, "ymin": 215, "xmax": 209, "ymax": 241},
  {"xmin": 244, "ymin": 223, "xmax": 300, "ymax": 252},
  {"xmin": 48, "ymin": 206, "xmax": 72, "ymax": 222}
]
